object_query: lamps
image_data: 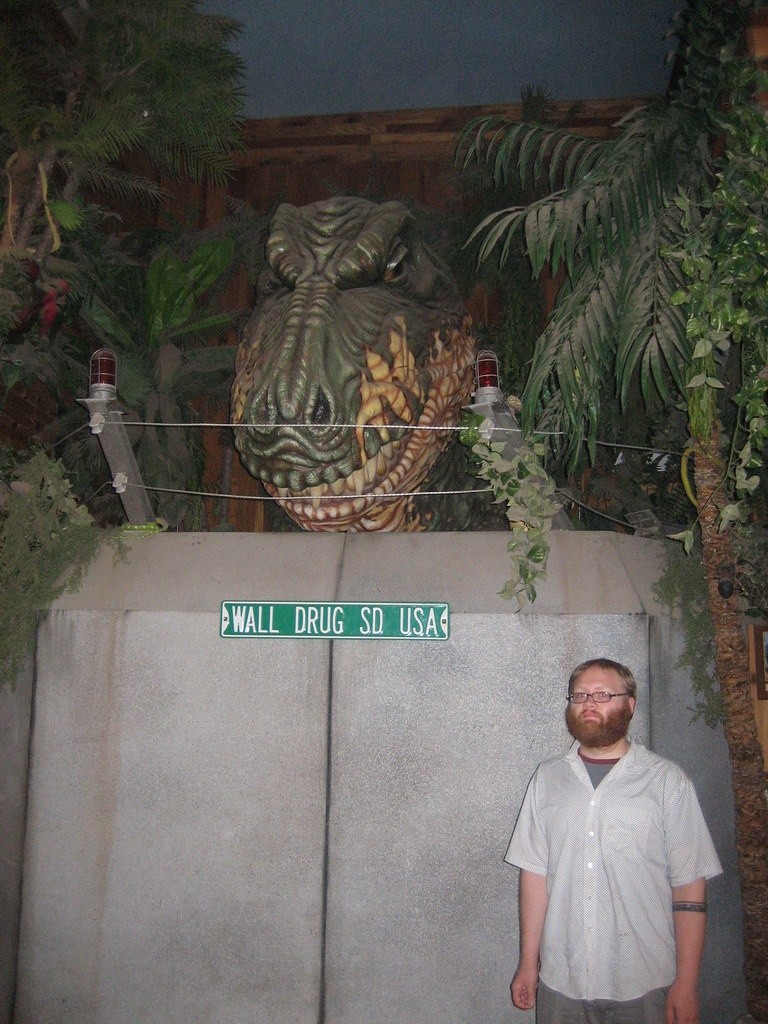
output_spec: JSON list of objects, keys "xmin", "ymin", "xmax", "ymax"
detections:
[
  {"xmin": 473, "ymin": 350, "xmax": 504, "ymax": 401},
  {"xmin": 87, "ymin": 350, "xmax": 121, "ymax": 399}
]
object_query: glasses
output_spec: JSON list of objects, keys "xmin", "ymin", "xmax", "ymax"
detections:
[{"xmin": 566, "ymin": 691, "xmax": 628, "ymax": 704}]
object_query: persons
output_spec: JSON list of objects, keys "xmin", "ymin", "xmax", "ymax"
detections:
[{"xmin": 502, "ymin": 659, "xmax": 724, "ymax": 1023}]
